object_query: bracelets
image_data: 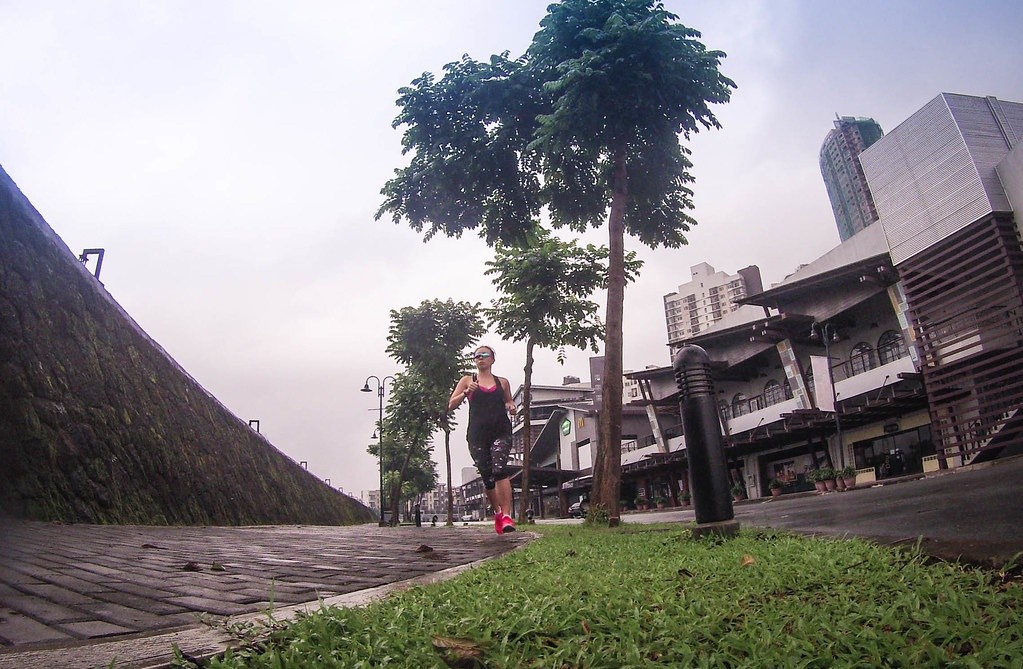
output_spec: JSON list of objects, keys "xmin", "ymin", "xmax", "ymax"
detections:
[{"xmin": 463, "ymin": 392, "xmax": 468, "ymax": 395}]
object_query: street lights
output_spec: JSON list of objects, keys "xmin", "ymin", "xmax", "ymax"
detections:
[
  {"xmin": 810, "ymin": 322, "xmax": 846, "ymax": 470},
  {"xmin": 361, "ymin": 375, "xmax": 399, "ymax": 530}
]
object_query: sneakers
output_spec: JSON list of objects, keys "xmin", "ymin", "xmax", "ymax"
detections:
[
  {"xmin": 503, "ymin": 515, "xmax": 517, "ymax": 532},
  {"xmin": 494, "ymin": 507, "xmax": 502, "ymax": 534}
]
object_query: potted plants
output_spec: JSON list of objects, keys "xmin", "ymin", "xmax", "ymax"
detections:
[
  {"xmin": 730, "ymin": 482, "xmax": 746, "ymax": 501},
  {"xmin": 654, "ymin": 496, "xmax": 668, "ymax": 509},
  {"xmin": 677, "ymin": 490, "xmax": 691, "ymax": 507},
  {"xmin": 634, "ymin": 497, "xmax": 649, "ymax": 510},
  {"xmin": 768, "ymin": 480, "xmax": 781, "ymax": 495},
  {"xmin": 812, "ymin": 465, "xmax": 857, "ymax": 491},
  {"xmin": 619, "ymin": 501, "xmax": 629, "ymax": 511}
]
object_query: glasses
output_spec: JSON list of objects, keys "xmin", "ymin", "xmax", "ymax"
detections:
[{"xmin": 475, "ymin": 352, "xmax": 490, "ymax": 358}]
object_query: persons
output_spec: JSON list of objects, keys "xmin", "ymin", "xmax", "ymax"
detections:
[{"xmin": 448, "ymin": 346, "xmax": 518, "ymax": 534}]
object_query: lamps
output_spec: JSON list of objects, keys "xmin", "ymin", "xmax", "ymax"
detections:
[
  {"xmin": 415, "ymin": 506, "xmax": 420, "ymax": 511},
  {"xmin": 679, "ymin": 362, "xmax": 710, "ymax": 400},
  {"xmin": 883, "ymin": 423, "xmax": 899, "ymax": 433}
]
